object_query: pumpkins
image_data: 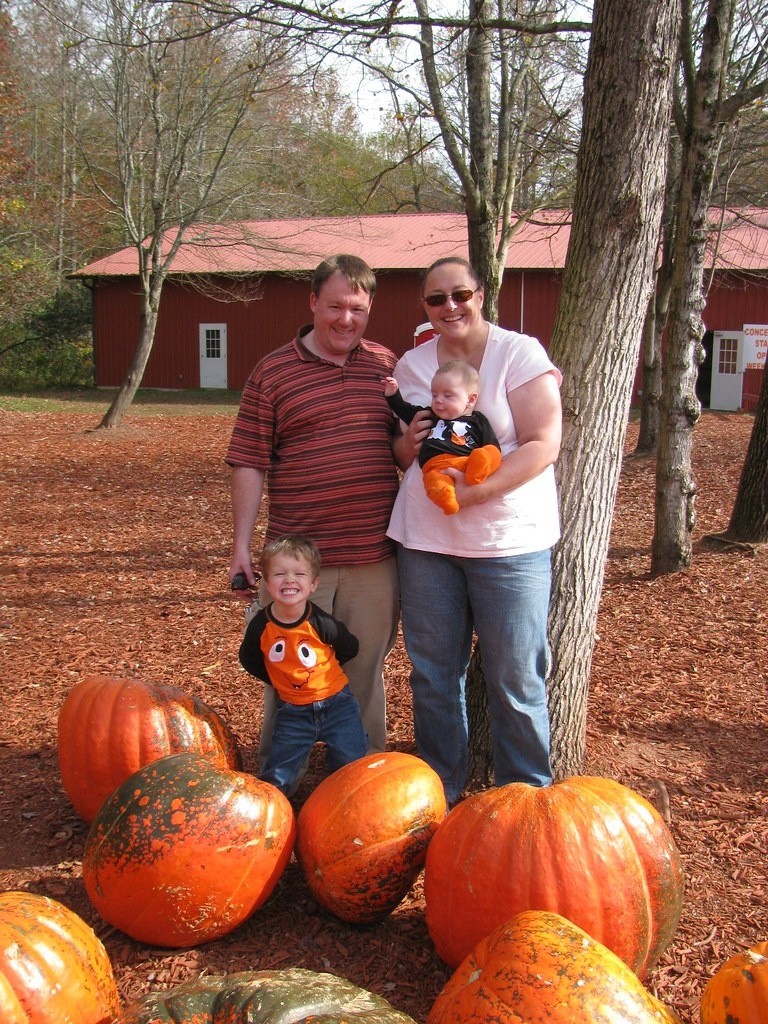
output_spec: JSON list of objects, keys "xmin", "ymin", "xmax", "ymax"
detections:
[
  {"xmin": 82, "ymin": 753, "xmax": 297, "ymax": 948},
  {"xmin": 103, "ymin": 967, "xmax": 419, "ymax": 1024},
  {"xmin": 423, "ymin": 776, "xmax": 684, "ymax": 986},
  {"xmin": 699, "ymin": 941, "xmax": 768, "ymax": 1024},
  {"xmin": 57, "ymin": 678, "xmax": 244, "ymax": 828},
  {"xmin": 417, "ymin": 910, "xmax": 688, "ymax": 1024},
  {"xmin": 293, "ymin": 751, "xmax": 446, "ymax": 926},
  {"xmin": 0, "ymin": 890, "xmax": 121, "ymax": 1024}
]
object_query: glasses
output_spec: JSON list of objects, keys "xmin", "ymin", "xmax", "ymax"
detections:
[
  {"xmin": 231, "ymin": 571, "xmax": 263, "ymax": 591},
  {"xmin": 424, "ymin": 286, "xmax": 479, "ymax": 306}
]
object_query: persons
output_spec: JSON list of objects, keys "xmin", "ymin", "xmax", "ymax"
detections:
[
  {"xmin": 380, "ymin": 358, "xmax": 503, "ymax": 515},
  {"xmin": 384, "ymin": 256, "xmax": 563, "ymax": 805},
  {"xmin": 225, "ymin": 252, "xmax": 400, "ymax": 799},
  {"xmin": 238, "ymin": 532, "xmax": 368, "ymax": 800}
]
